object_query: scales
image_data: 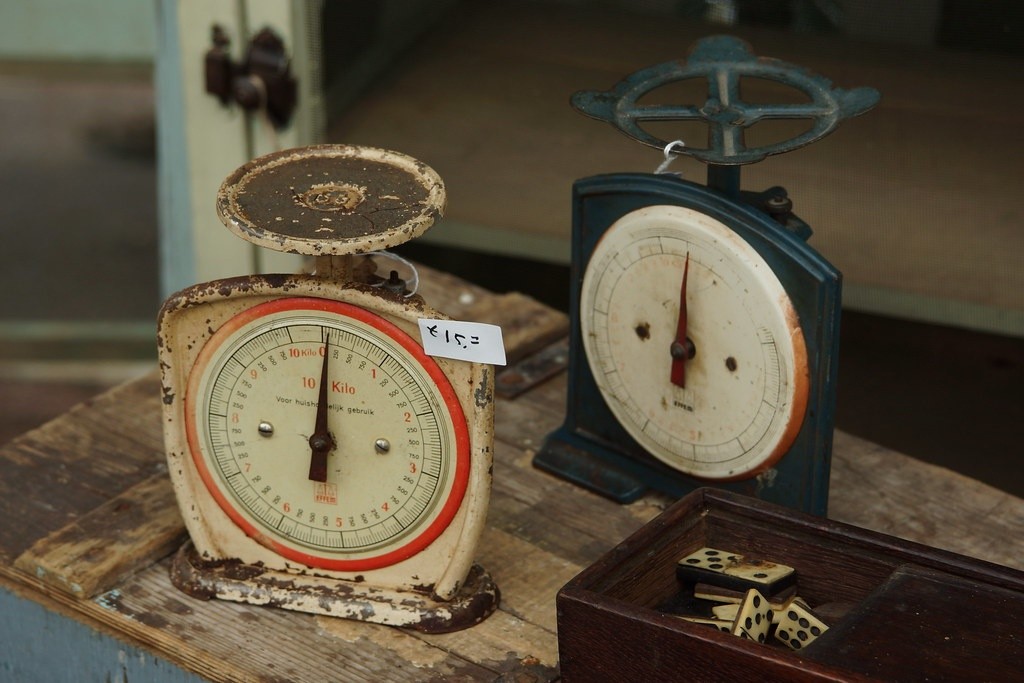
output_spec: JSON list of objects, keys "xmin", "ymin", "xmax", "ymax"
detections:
[
  {"xmin": 531, "ymin": 33, "xmax": 883, "ymax": 519},
  {"xmin": 154, "ymin": 144, "xmax": 500, "ymax": 634}
]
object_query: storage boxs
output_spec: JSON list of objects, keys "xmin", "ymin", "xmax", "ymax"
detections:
[{"xmin": 556, "ymin": 487, "xmax": 1024, "ymax": 683}]
